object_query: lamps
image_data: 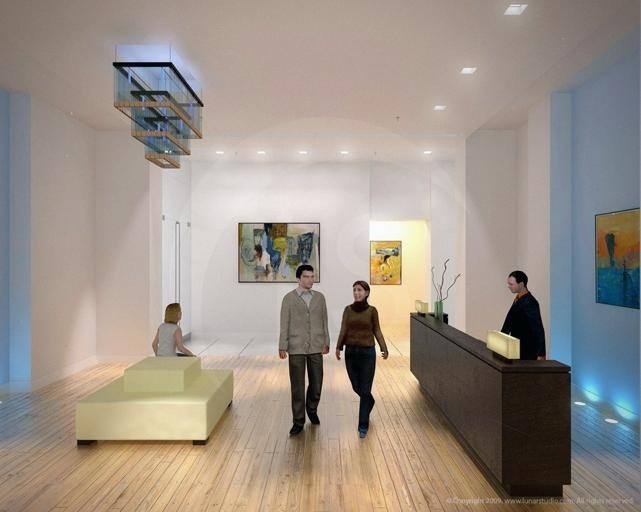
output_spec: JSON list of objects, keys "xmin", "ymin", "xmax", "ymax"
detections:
[{"xmin": 105, "ymin": 43, "xmax": 203, "ymax": 168}]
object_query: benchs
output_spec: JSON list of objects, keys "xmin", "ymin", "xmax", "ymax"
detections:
[{"xmin": 75, "ymin": 356, "xmax": 234, "ymax": 447}]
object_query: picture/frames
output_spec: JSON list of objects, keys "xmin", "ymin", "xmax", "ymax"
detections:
[
  {"xmin": 236, "ymin": 222, "xmax": 321, "ymax": 284},
  {"xmin": 593, "ymin": 206, "xmax": 640, "ymax": 310},
  {"xmin": 368, "ymin": 240, "xmax": 402, "ymax": 285}
]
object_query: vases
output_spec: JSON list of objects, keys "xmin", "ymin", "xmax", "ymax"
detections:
[{"xmin": 434, "ymin": 302, "xmax": 443, "ymax": 322}]
